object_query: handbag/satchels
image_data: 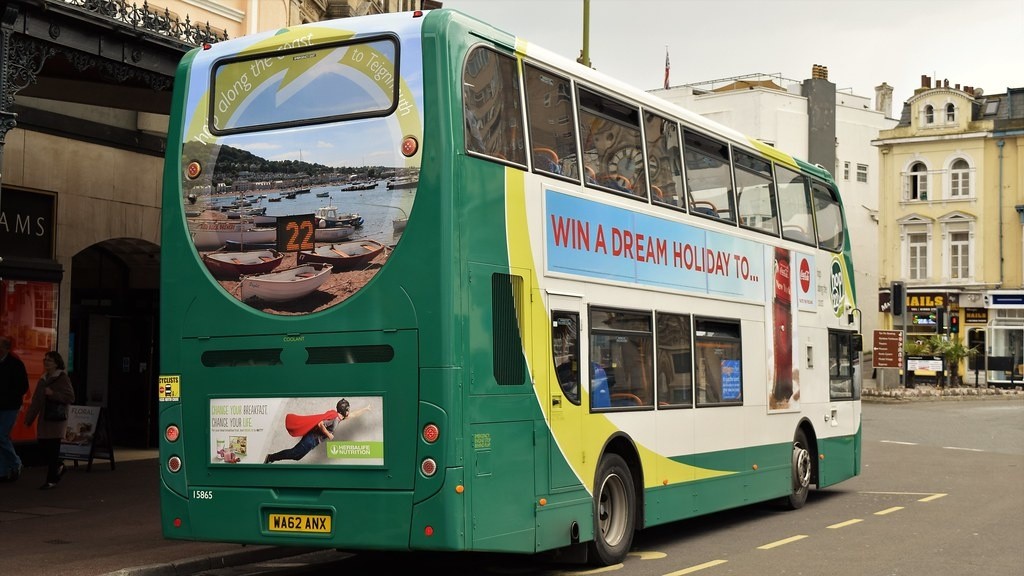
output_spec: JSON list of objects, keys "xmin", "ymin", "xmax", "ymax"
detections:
[{"xmin": 42, "ymin": 400, "xmax": 70, "ymax": 420}]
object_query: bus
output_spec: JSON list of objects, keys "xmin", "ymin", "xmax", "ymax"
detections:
[{"xmin": 159, "ymin": 10, "xmax": 863, "ymax": 566}]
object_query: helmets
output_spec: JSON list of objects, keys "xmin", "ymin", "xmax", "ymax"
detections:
[{"xmin": 336, "ymin": 399, "xmax": 349, "ymax": 417}]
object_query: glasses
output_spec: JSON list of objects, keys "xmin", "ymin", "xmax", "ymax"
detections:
[{"xmin": 42, "ymin": 358, "xmax": 53, "ymax": 362}]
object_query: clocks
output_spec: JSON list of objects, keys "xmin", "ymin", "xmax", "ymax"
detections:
[{"xmin": 606, "ymin": 144, "xmax": 659, "ymax": 193}]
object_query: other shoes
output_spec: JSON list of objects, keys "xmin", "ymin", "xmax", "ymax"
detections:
[
  {"xmin": 41, "ymin": 463, "xmax": 66, "ymax": 489},
  {"xmin": 7, "ymin": 460, "xmax": 23, "ymax": 482}
]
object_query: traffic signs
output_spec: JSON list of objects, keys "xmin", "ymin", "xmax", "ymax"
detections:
[{"xmin": 872, "ymin": 330, "xmax": 902, "ymax": 369}]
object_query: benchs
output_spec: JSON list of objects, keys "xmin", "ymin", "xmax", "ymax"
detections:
[
  {"xmin": 329, "ymin": 248, "xmax": 350, "ymax": 257},
  {"xmin": 231, "ymin": 258, "xmax": 241, "ymax": 264},
  {"xmin": 361, "ymin": 245, "xmax": 377, "ymax": 252},
  {"xmin": 295, "ymin": 273, "xmax": 316, "ymax": 278},
  {"xmin": 260, "ymin": 256, "xmax": 272, "ymax": 262}
]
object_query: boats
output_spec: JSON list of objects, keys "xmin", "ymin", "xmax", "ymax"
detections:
[
  {"xmin": 239, "ymin": 259, "xmax": 333, "ymax": 307},
  {"xmin": 341, "ymin": 181, "xmax": 378, "ymax": 191},
  {"xmin": 187, "ymin": 219, "xmax": 277, "ymax": 253},
  {"xmin": 277, "ymin": 213, "xmax": 384, "ymax": 272},
  {"xmin": 280, "ymin": 182, "xmax": 311, "ymax": 199},
  {"xmin": 317, "ymin": 192, "xmax": 330, "ymax": 198},
  {"xmin": 393, "ymin": 218, "xmax": 409, "ymax": 232},
  {"xmin": 268, "ymin": 197, "xmax": 282, "ymax": 202},
  {"xmin": 206, "ymin": 194, "xmax": 268, "ymax": 218},
  {"xmin": 387, "ymin": 175, "xmax": 419, "ymax": 189},
  {"xmin": 185, "ymin": 210, "xmax": 202, "ymax": 216},
  {"xmin": 203, "ymin": 248, "xmax": 284, "ymax": 278},
  {"xmin": 314, "ymin": 225, "xmax": 355, "ymax": 241},
  {"xmin": 315, "ymin": 197, "xmax": 365, "ymax": 228}
]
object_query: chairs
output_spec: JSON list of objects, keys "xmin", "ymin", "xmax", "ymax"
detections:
[
  {"xmin": 485, "ymin": 148, "xmax": 806, "ymax": 243},
  {"xmin": 557, "ymin": 360, "xmax": 669, "ymax": 407}
]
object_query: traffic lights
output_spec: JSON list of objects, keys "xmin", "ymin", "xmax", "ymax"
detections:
[
  {"xmin": 931, "ymin": 309, "xmax": 936, "ymax": 332},
  {"xmin": 950, "ymin": 316, "xmax": 959, "ymax": 333}
]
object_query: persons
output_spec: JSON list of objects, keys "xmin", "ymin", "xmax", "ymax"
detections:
[
  {"xmin": 264, "ymin": 398, "xmax": 372, "ymax": 464},
  {"xmin": 0, "ymin": 337, "xmax": 30, "ymax": 484},
  {"xmin": 24, "ymin": 351, "xmax": 75, "ymax": 490}
]
